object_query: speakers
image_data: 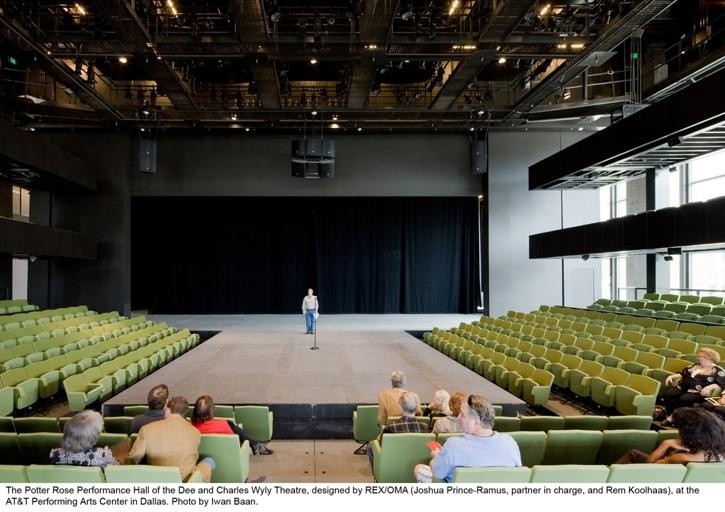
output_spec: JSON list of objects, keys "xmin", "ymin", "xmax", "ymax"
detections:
[
  {"xmin": 470, "ymin": 140, "xmax": 489, "ymax": 175},
  {"xmin": 291, "ymin": 138, "xmax": 336, "ymax": 179},
  {"xmin": 139, "ymin": 141, "xmax": 156, "ymax": 174}
]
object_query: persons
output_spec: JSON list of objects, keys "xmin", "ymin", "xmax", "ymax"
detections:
[
  {"xmin": 377, "ymin": 392, "xmax": 428, "ymax": 447},
  {"xmin": 44, "ymin": 410, "xmax": 122, "ymax": 480},
  {"xmin": 119, "ymin": 396, "xmax": 216, "ymax": 482},
  {"xmin": 432, "ymin": 391, "xmax": 465, "ymax": 433},
  {"xmin": 301, "ymin": 288, "xmax": 319, "ymax": 335},
  {"xmin": 423, "ymin": 389, "xmax": 454, "ymax": 429},
  {"xmin": 615, "ymin": 409, "xmax": 725, "ymax": 464},
  {"xmin": 377, "ymin": 371, "xmax": 423, "ymax": 425},
  {"xmin": 127, "ymin": 384, "xmax": 169, "ymax": 441},
  {"xmin": 190, "ymin": 394, "xmax": 273, "ymax": 456},
  {"xmin": 655, "ymin": 349, "xmax": 725, "ymax": 426},
  {"xmin": 413, "ymin": 395, "xmax": 521, "ymax": 483}
]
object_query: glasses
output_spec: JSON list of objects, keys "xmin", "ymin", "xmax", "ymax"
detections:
[{"xmin": 468, "ymin": 395, "xmax": 480, "ymax": 413}]
